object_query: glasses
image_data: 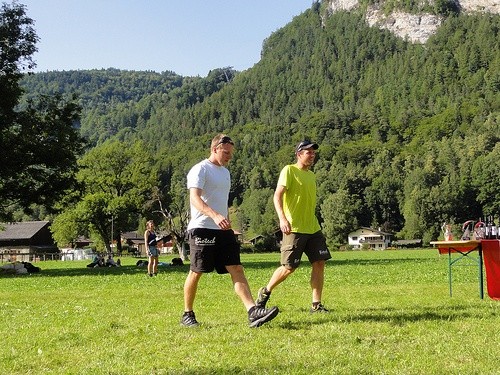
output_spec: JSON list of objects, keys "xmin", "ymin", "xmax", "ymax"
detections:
[
  {"xmin": 297, "ymin": 141, "xmax": 311, "ymax": 150},
  {"xmin": 215, "ymin": 136, "xmax": 234, "ymax": 148}
]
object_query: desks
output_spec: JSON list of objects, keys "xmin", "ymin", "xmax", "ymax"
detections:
[{"xmin": 430, "ymin": 237, "xmax": 500, "ymax": 299}]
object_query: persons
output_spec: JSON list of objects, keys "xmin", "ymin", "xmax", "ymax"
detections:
[
  {"xmin": 255, "ymin": 141, "xmax": 332, "ymax": 315},
  {"xmin": 180, "ymin": 134, "xmax": 279, "ymax": 328},
  {"xmin": 144, "ymin": 220, "xmax": 158, "ymax": 278},
  {"xmin": 87, "ymin": 255, "xmax": 121, "ymax": 268}
]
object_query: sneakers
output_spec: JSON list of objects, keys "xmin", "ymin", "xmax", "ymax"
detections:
[
  {"xmin": 248, "ymin": 305, "xmax": 279, "ymax": 328},
  {"xmin": 255, "ymin": 286, "xmax": 269, "ymax": 310},
  {"xmin": 312, "ymin": 303, "xmax": 329, "ymax": 313},
  {"xmin": 182, "ymin": 313, "xmax": 198, "ymax": 327}
]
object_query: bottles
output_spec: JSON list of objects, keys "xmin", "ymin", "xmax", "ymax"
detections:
[{"xmin": 483, "ymin": 216, "xmax": 500, "ymax": 240}]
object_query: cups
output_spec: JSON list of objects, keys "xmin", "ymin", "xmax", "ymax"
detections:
[{"xmin": 444, "ymin": 233, "xmax": 449, "ymax": 241}]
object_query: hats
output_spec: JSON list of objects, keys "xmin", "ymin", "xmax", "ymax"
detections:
[{"xmin": 295, "ymin": 141, "xmax": 319, "ymax": 154}]
